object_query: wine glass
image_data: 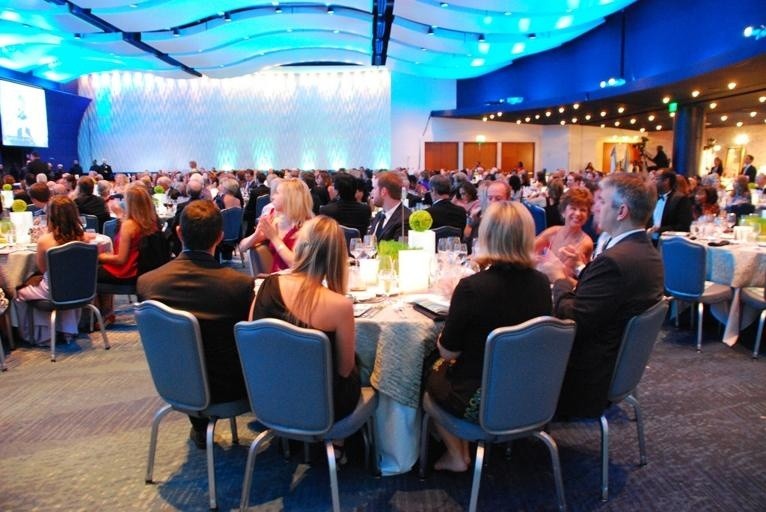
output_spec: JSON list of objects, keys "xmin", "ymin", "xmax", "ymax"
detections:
[
  {"xmin": 151, "ymin": 197, "xmax": 176, "ymax": 216},
  {"xmin": 346, "ymin": 235, "xmax": 470, "ymax": 291},
  {"xmin": 1, "ymin": 214, "xmax": 49, "ymax": 247},
  {"xmin": 693, "ymin": 216, "xmax": 764, "ymax": 244}
]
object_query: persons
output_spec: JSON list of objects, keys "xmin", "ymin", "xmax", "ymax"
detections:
[
  {"xmin": 136, "ymin": 199, "xmax": 296, "ymax": 456},
  {"xmin": 420, "ymin": 200, "xmax": 552, "ymax": 477},
  {"xmin": 633, "ymin": 145, "xmax": 766, "ymax": 249},
  {"xmin": 551, "ymin": 173, "xmax": 664, "ymax": 417},
  {"xmin": 248, "ymin": 214, "xmax": 361, "ymax": 472}
]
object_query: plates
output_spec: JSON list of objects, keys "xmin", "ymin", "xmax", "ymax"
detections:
[{"xmin": 351, "ymin": 303, "xmax": 374, "ymax": 318}]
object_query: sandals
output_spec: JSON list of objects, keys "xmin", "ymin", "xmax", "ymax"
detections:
[{"xmin": 328, "ymin": 446, "xmax": 350, "ymax": 473}]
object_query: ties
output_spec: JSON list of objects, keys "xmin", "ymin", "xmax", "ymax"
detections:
[{"xmin": 375, "ymin": 214, "xmax": 387, "ymax": 238}]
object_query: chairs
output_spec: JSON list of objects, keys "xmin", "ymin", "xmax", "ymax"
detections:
[
  {"xmin": 1, "ymin": 299, "xmax": 18, "ymax": 372},
  {"xmin": 557, "ymin": 296, "xmax": 671, "ymax": 502},
  {"xmin": 256, "ymin": 195, "xmax": 274, "ymax": 219},
  {"xmin": 23, "ymin": 241, "xmax": 112, "ymax": 363},
  {"xmin": 415, "ymin": 315, "xmax": 577, "ymax": 509},
  {"xmin": 131, "ymin": 301, "xmax": 247, "ymax": 511},
  {"xmin": 234, "ymin": 317, "xmax": 382, "ymax": 511},
  {"xmin": 219, "ymin": 207, "xmax": 248, "ymax": 268},
  {"xmin": 661, "ymin": 236, "xmax": 732, "ymax": 353},
  {"xmin": 96, "ymin": 233, "xmax": 171, "ymax": 308},
  {"xmin": 741, "ymin": 286, "xmax": 766, "ymax": 360}
]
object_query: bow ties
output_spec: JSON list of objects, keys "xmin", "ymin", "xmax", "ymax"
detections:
[{"xmin": 657, "ymin": 195, "xmax": 665, "ymax": 201}]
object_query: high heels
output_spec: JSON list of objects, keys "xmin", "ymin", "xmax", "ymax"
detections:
[{"xmin": 95, "ymin": 313, "xmax": 116, "ymax": 331}]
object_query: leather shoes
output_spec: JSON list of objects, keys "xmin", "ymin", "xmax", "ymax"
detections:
[{"xmin": 189, "ymin": 428, "xmax": 206, "ymax": 449}]
